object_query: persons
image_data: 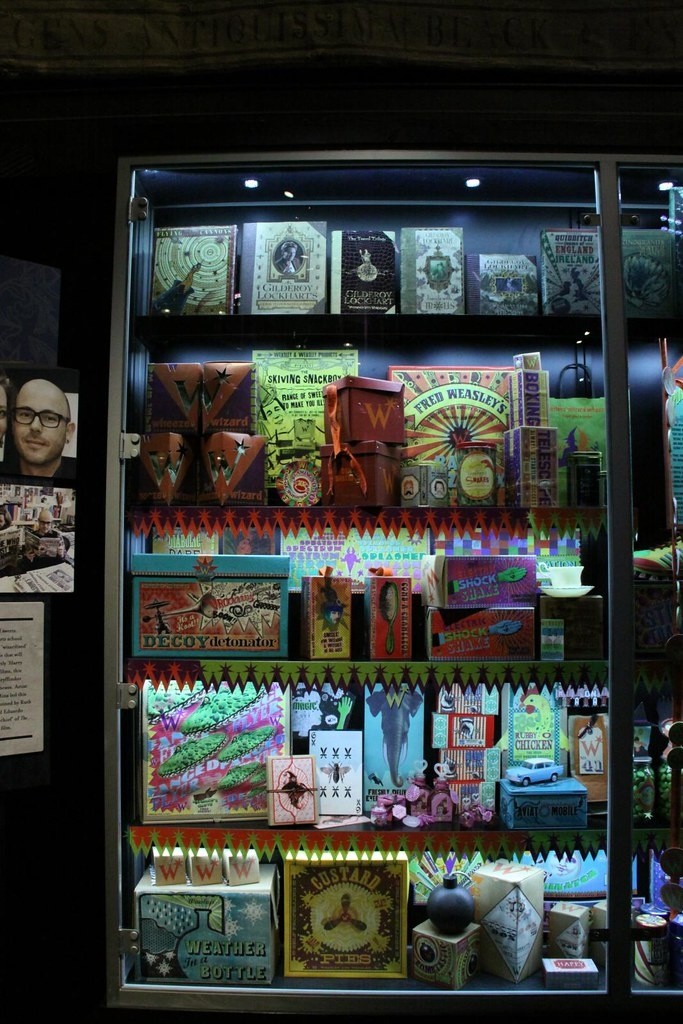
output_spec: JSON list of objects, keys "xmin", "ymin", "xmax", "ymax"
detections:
[
  {"xmin": 0, "ymin": 508, "xmax": 12, "ymax": 531},
  {"xmin": 0, "ymin": 376, "xmax": 73, "ymax": 478},
  {"xmin": 18, "ymin": 510, "xmax": 65, "ymax": 572}
]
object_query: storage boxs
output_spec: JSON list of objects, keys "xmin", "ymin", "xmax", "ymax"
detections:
[{"xmin": 132, "ymin": 220, "xmax": 607, "ymax": 987}]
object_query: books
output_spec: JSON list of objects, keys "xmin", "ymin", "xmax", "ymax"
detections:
[{"xmin": 25, "ymin": 532, "xmax": 60, "ymax": 558}]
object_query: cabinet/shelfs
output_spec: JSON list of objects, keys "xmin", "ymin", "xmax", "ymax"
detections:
[
  {"xmin": 627, "ymin": 317, "xmax": 683, "ymax": 862},
  {"xmin": 129, "ymin": 314, "xmax": 609, "ymax": 858}
]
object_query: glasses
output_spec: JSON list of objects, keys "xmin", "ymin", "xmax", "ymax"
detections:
[
  {"xmin": 10, "ymin": 407, "xmax": 67, "ymax": 428},
  {"xmin": 38, "ymin": 519, "xmax": 50, "ymax": 524}
]
